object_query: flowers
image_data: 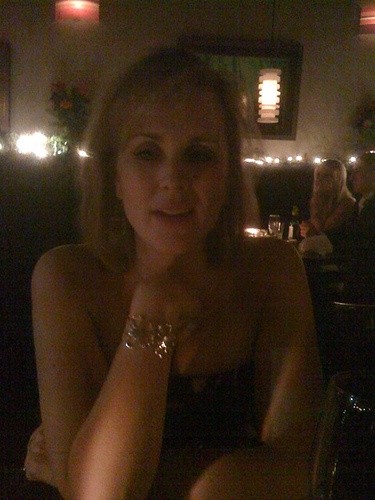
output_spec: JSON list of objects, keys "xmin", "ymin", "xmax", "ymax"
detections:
[
  {"xmin": 354, "ymin": 94, "xmax": 374, "ymax": 150},
  {"xmin": 44, "ymin": 80, "xmax": 92, "ymax": 156}
]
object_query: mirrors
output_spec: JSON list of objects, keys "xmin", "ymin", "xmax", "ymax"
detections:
[{"xmin": 178, "ymin": 34, "xmax": 302, "ymax": 142}]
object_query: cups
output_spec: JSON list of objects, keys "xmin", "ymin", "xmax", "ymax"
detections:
[
  {"xmin": 311, "ymin": 370, "xmax": 375, "ymax": 500},
  {"xmin": 268, "ymin": 215, "xmax": 281, "ymax": 235}
]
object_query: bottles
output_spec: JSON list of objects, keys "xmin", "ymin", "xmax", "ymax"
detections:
[{"xmin": 290, "ymin": 206, "xmax": 299, "ymax": 239}]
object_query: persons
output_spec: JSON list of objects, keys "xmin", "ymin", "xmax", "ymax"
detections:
[
  {"xmin": 26, "ymin": 48, "xmax": 326, "ymax": 500},
  {"xmin": 301, "ymin": 153, "xmax": 375, "ymax": 330}
]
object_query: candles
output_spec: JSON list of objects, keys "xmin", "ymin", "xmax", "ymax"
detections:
[{"xmin": 288, "ymin": 224, "xmax": 293, "ymax": 237}]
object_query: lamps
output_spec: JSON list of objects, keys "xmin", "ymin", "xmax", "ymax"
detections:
[
  {"xmin": 55, "ymin": 1, "xmax": 100, "ymax": 22},
  {"xmin": 359, "ymin": 3, "xmax": 375, "ymax": 41},
  {"xmin": 257, "ymin": 69, "xmax": 281, "ymax": 123}
]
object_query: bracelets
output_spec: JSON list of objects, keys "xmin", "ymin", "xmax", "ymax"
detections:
[{"xmin": 121, "ymin": 318, "xmax": 176, "ymax": 359}]
